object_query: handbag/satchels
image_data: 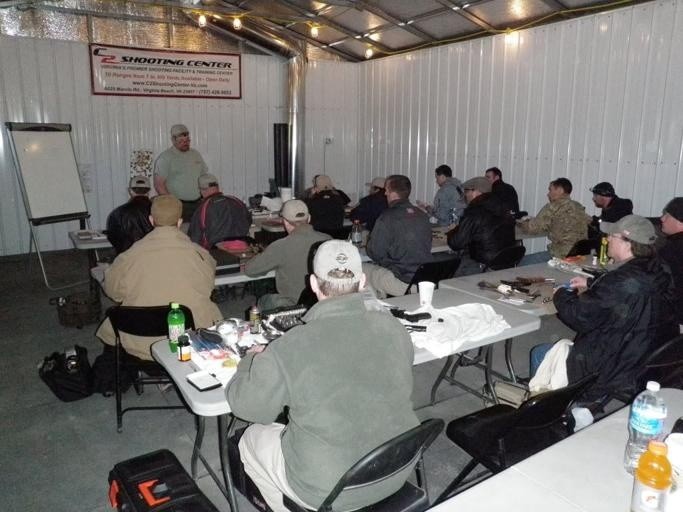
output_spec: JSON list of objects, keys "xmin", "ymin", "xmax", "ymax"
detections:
[
  {"xmin": 91, "ymin": 344, "xmax": 140, "ymax": 397},
  {"xmin": 49, "ymin": 291, "xmax": 101, "ymax": 329},
  {"xmin": 35, "ymin": 344, "xmax": 100, "ymax": 402},
  {"xmin": 108, "ymin": 449, "xmax": 220, "ymax": 512}
]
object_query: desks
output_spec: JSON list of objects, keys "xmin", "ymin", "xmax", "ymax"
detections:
[
  {"xmin": 149, "ymin": 287, "xmax": 542, "ymax": 511},
  {"xmin": 425, "ymin": 387, "xmax": 683, "ymax": 512},
  {"xmin": 562, "ymin": 254, "xmax": 603, "ymax": 275},
  {"xmin": 437, "ymin": 262, "xmax": 596, "ymax": 393}
]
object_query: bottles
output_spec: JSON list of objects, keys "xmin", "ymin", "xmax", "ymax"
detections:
[
  {"xmin": 631, "ymin": 438, "xmax": 671, "ymax": 511},
  {"xmin": 248, "ymin": 305, "xmax": 260, "ymax": 336},
  {"xmin": 166, "ymin": 303, "xmax": 184, "ymax": 351},
  {"xmin": 599, "ymin": 238, "xmax": 608, "ymax": 265},
  {"xmin": 624, "ymin": 381, "xmax": 666, "ymax": 475},
  {"xmin": 176, "ymin": 335, "xmax": 191, "ymax": 364},
  {"xmin": 592, "ymin": 255, "xmax": 597, "ymax": 265},
  {"xmin": 449, "ymin": 207, "xmax": 458, "ymax": 227},
  {"xmin": 351, "ymin": 220, "xmax": 362, "ymax": 249}
]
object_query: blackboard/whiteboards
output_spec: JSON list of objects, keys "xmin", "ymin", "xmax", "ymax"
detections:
[{"xmin": 7, "ymin": 123, "xmax": 91, "ymax": 225}]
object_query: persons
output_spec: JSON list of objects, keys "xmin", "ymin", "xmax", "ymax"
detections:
[
  {"xmin": 99, "ymin": 176, "xmax": 155, "ymax": 263},
  {"xmin": 186, "ymin": 163, "xmax": 683, "ymax": 412},
  {"xmin": 153, "ymin": 124, "xmax": 209, "ymax": 224},
  {"xmin": 97, "ymin": 196, "xmax": 224, "ymax": 393},
  {"xmin": 225, "ymin": 237, "xmax": 422, "ymax": 512}
]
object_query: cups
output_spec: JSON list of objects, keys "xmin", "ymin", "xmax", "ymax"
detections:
[
  {"xmin": 418, "ymin": 281, "xmax": 435, "ymax": 306},
  {"xmin": 280, "ymin": 188, "xmax": 290, "ymax": 202}
]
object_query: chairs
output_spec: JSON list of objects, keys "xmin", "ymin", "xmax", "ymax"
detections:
[
  {"xmin": 591, "ymin": 333, "xmax": 683, "ymax": 417},
  {"xmin": 317, "ymin": 419, "xmax": 445, "ymax": 511},
  {"xmin": 565, "ymin": 239, "xmax": 601, "ymax": 258},
  {"xmin": 425, "ymin": 372, "xmax": 600, "ymax": 512},
  {"xmin": 108, "ymin": 304, "xmax": 196, "ymax": 433},
  {"xmin": 296, "ymin": 238, "xmax": 333, "ymax": 318}
]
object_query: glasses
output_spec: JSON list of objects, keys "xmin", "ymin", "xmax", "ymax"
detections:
[{"xmin": 177, "ymin": 139, "xmax": 191, "ymax": 144}]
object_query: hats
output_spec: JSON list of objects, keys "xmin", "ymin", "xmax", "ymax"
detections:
[
  {"xmin": 312, "ymin": 239, "xmax": 363, "ymax": 284},
  {"xmin": 365, "ymin": 177, "xmax": 386, "ymax": 189},
  {"xmin": 279, "ymin": 199, "xmax": 309, "ymax": 222},
  {"xmin": 599, "ymin": 215, "xmax": 658, "ymax": 245},
  {"xmin": 130, "ymin": 175, "xmax": 151, "ymax": 188},
  {"xmin": 198, "ymin": 174, "xmax": 218, "ymax": 189},
  {"xmin": 170, "ymin": 125, "xmax": 189, "ymax": 137},
  {"xmin": 589, "ymin": 182, "xmax": 615, "ymax": 197},
  {"xmin": 460, "ymin": 177, "xmax": 492, "ymax": 194},
  {"xmin": 150, "ymin": 194, "xmax": 183, "ymax": 226},
  {"xmin": 664, "ymin": 197, "xmax": 683, "ymax": 223}
]
